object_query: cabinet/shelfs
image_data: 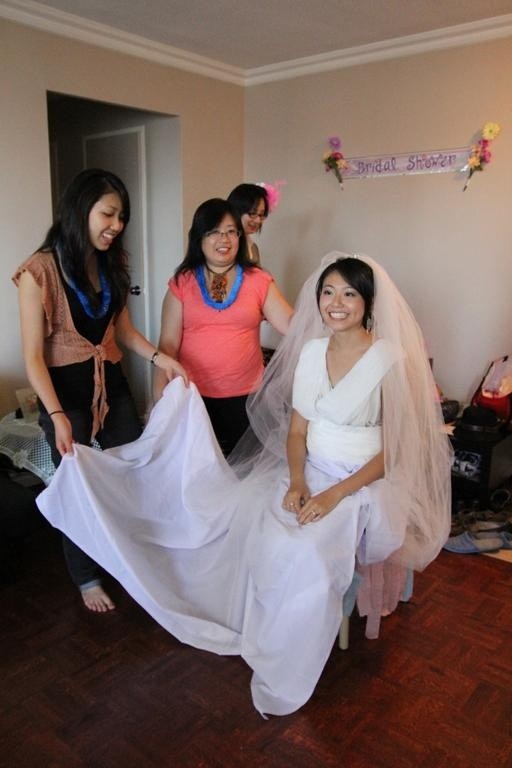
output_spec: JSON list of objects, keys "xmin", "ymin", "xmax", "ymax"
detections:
[{"xmin": 446, "ymin": 420, "xmax": 512, "ymax": 507}]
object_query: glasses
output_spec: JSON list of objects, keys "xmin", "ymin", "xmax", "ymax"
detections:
[
  {"xmin": 247, "ymin": 211, "xmax": 266, "ymax": 220},
  {"xmin": 205, "ymin": 227, "xmax": 242, "ymax": 239}
]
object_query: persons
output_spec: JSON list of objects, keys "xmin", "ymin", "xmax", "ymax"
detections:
[
  {"xmin": 146, "ymin": 195, "xmax": 317, "ymax": 453},
  {"xmin": 34, "ymin": 249, "xmax": 460, "ymax": 720},
  {"xmin": 10, "ymin": 167, "xmax": 194, "ymax": 613},
  {"xmin": 223, "ymin": 183, "xmax": 272, "ymax": 277}
]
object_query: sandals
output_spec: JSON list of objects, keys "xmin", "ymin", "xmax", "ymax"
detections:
[{"xmin": 488, "ymin": 476, "xmax": 512, "ymax": 513}]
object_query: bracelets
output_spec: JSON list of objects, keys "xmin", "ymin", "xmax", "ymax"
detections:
[
  {"xmin": 151, "ymin": 351, "xmax": 161, "ymax": 368},
  {"xmin": 48, "ymin": 411, "xmax": 65, "ymax": 416}
]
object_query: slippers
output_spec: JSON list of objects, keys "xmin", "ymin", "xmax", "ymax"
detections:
[{"xmin": 443, "ymin": 511, "xmax": 512, "ymax": 553}]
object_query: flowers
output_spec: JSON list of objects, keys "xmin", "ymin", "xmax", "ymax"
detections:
[
  {"xmin": 459, "ymin": 122, "xmax": 500, "ymax": 191},
  {"xmin": 322, "ymin": 137, "xmax": 351, "ymax": 192}
]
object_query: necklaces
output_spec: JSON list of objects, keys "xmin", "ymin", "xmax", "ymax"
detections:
[{"xmin": 202, "ymin": 261, "xmax": 237, "ymax": 304}]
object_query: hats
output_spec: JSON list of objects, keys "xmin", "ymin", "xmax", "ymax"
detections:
[{"xmin": 452, "ymin": 406, "xmax": 503, "ymax": 442}]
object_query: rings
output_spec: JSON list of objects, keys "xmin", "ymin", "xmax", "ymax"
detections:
[
  {"xmin": 289, "ymin": 502, "xmax": 294, "ymax": 506},
  {"xmin": 312, "ymin": 510, "xmax": 316, "ymax": 516}
]
